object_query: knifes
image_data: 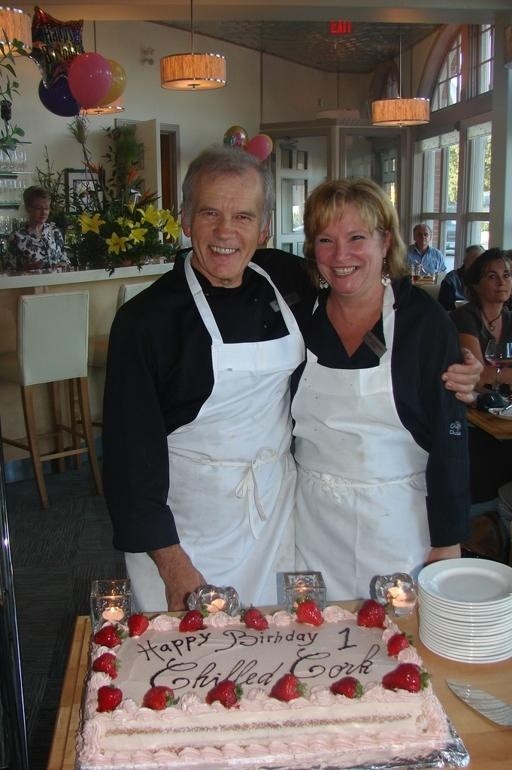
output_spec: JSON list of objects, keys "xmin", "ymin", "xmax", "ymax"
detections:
[{"xmin": 446, "ymin": 677, "xmax": 512, "ymax": 727}]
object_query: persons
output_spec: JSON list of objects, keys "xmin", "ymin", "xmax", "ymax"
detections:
[
  {"xmin": 102, "ymin": 145, "xmax": 485, "ymax": 616},
  {"xmin": 6, "ymin": 185, "xmax": 71, "ymax": 273},
  {"xmin": 448, "ymin": 247, "xmax": 511, "ymax": 386},
  {"xmin": 290, "ymin": 178, "xmax": 474, "ymax": 604},
  {"xmin": 438, "ymin": 244, "xmax": 486, "ymax": 312},
  {"xmin": 404, "ymin": 224, "xmax": 447, "ymax": 279}
]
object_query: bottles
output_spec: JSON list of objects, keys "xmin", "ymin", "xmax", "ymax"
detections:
[{"xmin": 0, "ymin": 241, "xmax": 8, "ymax": 256}]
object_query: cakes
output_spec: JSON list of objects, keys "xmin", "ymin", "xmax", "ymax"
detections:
[{"xmin": 74, "ymin": 604, "xmax": 454, "ymax": 770}]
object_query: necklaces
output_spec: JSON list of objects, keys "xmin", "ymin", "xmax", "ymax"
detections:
[{"xmin": 481, "ymin": 307, "xmax": 503, "ymax": 331}]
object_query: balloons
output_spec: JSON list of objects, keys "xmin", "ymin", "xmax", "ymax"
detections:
[
  {"xmin": 91, "ymin": 58, "xmax": 127, "ymax": 109},
  {"xmin": 223, "ymin": 126, "xmax": 248, "ymax": 152},
  {"xmin": 38, "ymin": 73, "xmax": 81, "ymax": 117},
  {"xmin": 68, "ymin": 52, "xmax": 112, "ymax": 112},
  {"xmin": 246, "ymin": 134, "xmax": 273, "ymax": 162},
  {"xmin": 29, "ymin": 5, "xmax": 85, "ymax": 90}
]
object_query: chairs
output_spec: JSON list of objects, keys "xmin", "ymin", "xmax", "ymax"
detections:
[
  {"xmin": 0, "ymin": 290, "xmax": 103, "ymax": 506},
  {"xmin": 68, "ymin": 280, "xmax": 157, "ymax": 471}
]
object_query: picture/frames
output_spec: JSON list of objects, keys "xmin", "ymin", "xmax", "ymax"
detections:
[{"xmin": 65, "ymin": 168, "xmax": 107, "ymax": 215}]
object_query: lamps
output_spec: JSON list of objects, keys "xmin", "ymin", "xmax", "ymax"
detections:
[
  {"xmin": 371, "ymin": 25, "xmax": 431, "ymax": 127},
  {"xmin": 79, "ymin": 21, "xmax": 127, "ymax": 116},
  {"xmin": 160, "ymin": 0, "xmax": 227, "ymax": 91},
  {"xmin": 0, "ymin": 7, "xmax": 33, "ymax": 59}
]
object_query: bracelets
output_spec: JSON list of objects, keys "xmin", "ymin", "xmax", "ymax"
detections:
[{"xmin": 495, "ymin": 367, "xmax": 501, "ymax": 386}]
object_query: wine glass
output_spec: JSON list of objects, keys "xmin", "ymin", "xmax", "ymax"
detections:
[
  {"xmin": 0, "ymin": 150, "xmax": 26, "ymax": 173},
  {"xmin": 412, "ymin": 267, "xmax": 420, "ymax": 285},
  {"xmin": 0, "ymin": 215, "xmax": 26, "ymax": 234},
  {"xmin": 0, "ymin": 180, "xmax": 28, "ymax": 203},
  {"xmin": 485, "ymin": 339, "xmax": 512, "ymax": 397}
]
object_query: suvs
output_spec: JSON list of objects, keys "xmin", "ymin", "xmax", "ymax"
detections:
[{"xmin": 444, "ymin": 205, "xmax": 457, "ymax": 253}]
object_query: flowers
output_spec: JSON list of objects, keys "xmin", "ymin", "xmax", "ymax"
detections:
[{"xmin": 66, "ymin": 113, "xmax": 182, "ymax": 277}]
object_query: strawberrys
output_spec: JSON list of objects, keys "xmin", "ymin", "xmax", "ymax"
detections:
[
  {"xmin": 381, "ymin": 663, "xmax": 434, "ymax": 694},
  {"xmin": 96, "ymin": 685, "xmax": 124, "ymax": 714},
  {"xmin": 240, "ymin": 608, "xmax": 269, "ymax": 632},
  {"xmin": 356, "ymin": 599, "xmax": 387, "ymax": 629},
  {"xmin": 93, "ymin": 623, "xmax": 126, "ymax": 649},
  {"xmin": 139, "ymin": 685, "xmax": 180, "ymax": 713},
  {"xmin": 127, "ymin": 612, "xmax": 150, "ymax": 639},
  {"xmin": 329, "ymin": 676, "xmax": 364, "ymax": 699},
  {"xmin": 205, "ymin": 679, "xmax": 243, "ymax": 711},
  {"xmin": 268, "ymin": 672, "xmax": 309, "ymax": 704},
  {"xmin": 291, "ymin": 598, "xmax": 325, "ymax": 627},
  {"xmin": 385, "ymin": 631, "xmax": 416, "ymax": 657},
  {"xmin": 178, "ymin": 609, "xmax": 209, "ymax": 633},
  {"xmin": 92, "ymin": 652, "xmax": 122, "ymax": 681}
]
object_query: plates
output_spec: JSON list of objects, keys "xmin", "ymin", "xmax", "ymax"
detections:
[{"xmin": 418, "ymin": 557, "xmax": 512, "ymax": 665}]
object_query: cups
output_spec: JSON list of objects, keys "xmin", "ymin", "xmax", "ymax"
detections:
[
  {"xmin": 375, "ymin": 574, "xmax": 414, "ymax": 609},
  {"xmin": 89, "ymin": 579, "xmax": 132, "ymax": 627},
  {"xmin": 284, "ymin": 570, "xmax": 327, "ymax": 615},
  {"xmin": 432, "ymin": 271, "xmax": 439, "ymax": 284},
  {"xmin": 185, "ymin": 585, "xmax": 241, "ymax": 615}
]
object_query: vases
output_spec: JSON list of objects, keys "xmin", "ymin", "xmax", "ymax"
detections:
[{"xmin": 96, "ymin": 255, "xmax": 144, "ymax": 268}]
